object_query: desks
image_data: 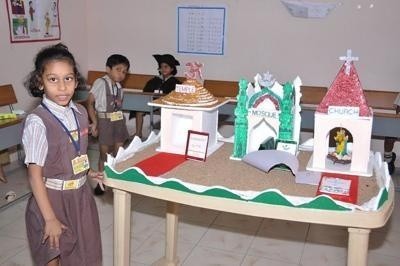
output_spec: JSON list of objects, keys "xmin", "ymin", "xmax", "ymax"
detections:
[
  {"xmin": 99, "ymin": 128, "xmax": 396, "ymax": 266},
  {"xmin": 1, "ymin": 112, "xmax": 30, "ymax": 184},
  {"xmin": 70, "ymin": 87, "xmax": 399, "ymax": 141}
]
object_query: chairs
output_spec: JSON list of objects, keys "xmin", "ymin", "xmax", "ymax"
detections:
[{"xmin": 0, "ymin": 84, "xmax": 24, "ymax": 123}]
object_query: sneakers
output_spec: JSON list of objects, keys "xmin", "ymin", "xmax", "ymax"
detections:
[
  {"xmin": 95, "ymin": 183, "xmax": 105, "ymax": 196},
  {"xmin": 385, "ymin": 153, "xmax": 396, "ymax": 175}
]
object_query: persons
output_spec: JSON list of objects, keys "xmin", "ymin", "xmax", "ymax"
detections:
[
  {"xmin": 130, "ymin": 54, "xmax": 181, "ymax": 140},
  {"xmin": 21, "ymin": 43, "xmax": 105, "ymax": 266},
  {"xmin": 87, "ymin": 53, "xmax": 130, "ymax": 195}
]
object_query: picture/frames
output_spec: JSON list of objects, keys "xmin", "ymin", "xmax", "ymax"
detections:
[
  {"xmin": 175, "ymin": 4, "xmax": 229, "ymax": 57},
  {"xmin": 5, "ymin": 0, "xmax": 62, "ymax": 43}
]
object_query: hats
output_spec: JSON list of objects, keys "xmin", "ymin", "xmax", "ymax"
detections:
[{"xmin": 152, "ymin": 54, "xmax": 180, "ymax": 67}]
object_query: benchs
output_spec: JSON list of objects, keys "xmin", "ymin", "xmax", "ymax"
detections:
[{"xmin": 88, "ymin": 69, "xmax": 400, "ymax": 118}]
